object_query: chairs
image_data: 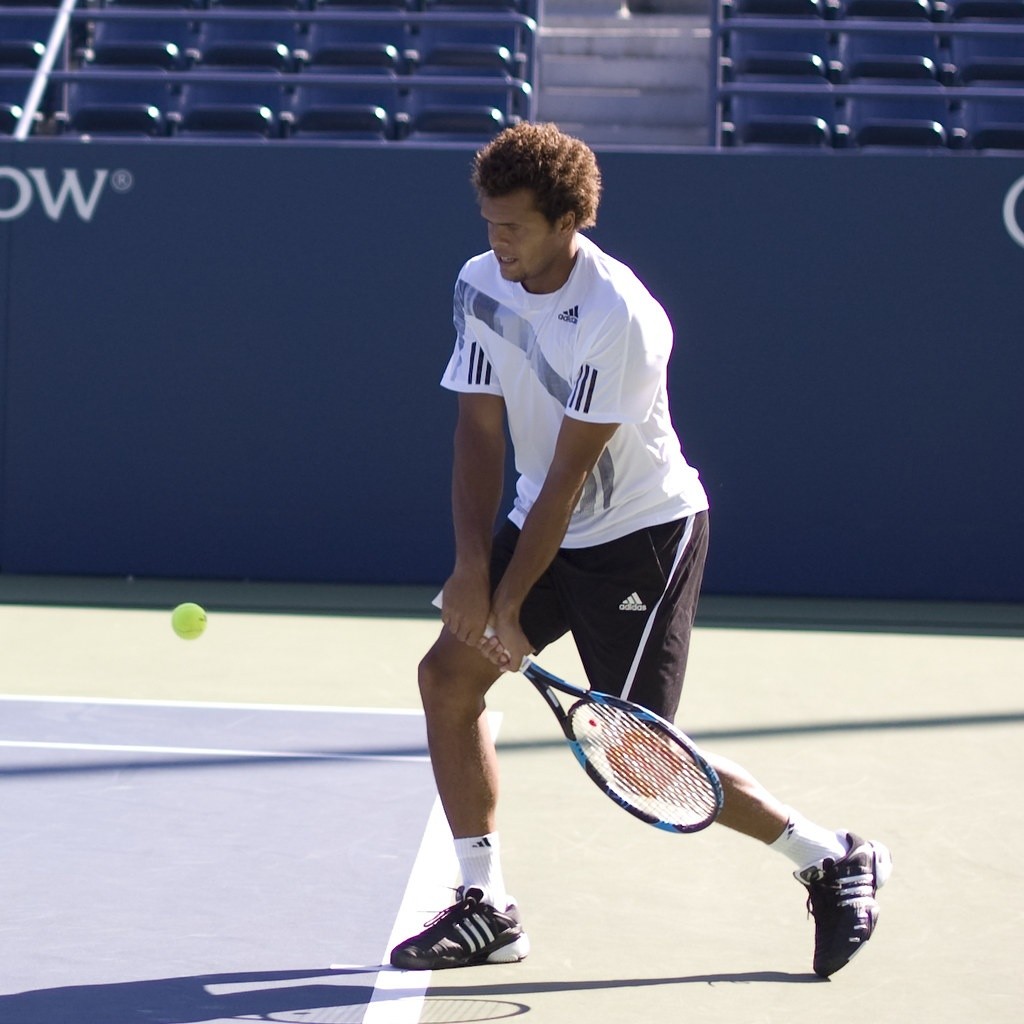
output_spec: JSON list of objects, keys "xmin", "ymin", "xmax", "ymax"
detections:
[
  {"xmin": 711, "ymin": 1, "xmax": 1023, "ymax": 149},
  {"xmin": 0, "ymin": 1, "xmax": 537, "ymax": 142}
]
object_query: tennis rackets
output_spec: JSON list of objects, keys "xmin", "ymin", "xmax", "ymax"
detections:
[{"xmin": 430, "ymin": 587, "xmax": 722, "ymax": 835}]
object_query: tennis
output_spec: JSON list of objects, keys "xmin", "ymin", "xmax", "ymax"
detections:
[{"xmin": 171, "ymin": 602, "xmax": 208, "ymax": 640}]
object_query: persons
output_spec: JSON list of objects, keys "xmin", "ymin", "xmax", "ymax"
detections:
[{"xmin": 386, "ymin": 124, "xmax": 892, "ymax": 977}]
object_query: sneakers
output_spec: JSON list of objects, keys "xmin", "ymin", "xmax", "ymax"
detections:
[
  {"xmin": 793, "ymin": 829, "xmax": 893, "ymax": 977},
  {"xmin": 390, "ymin": 885, "xmax": 530, "ymax": 970}
]
